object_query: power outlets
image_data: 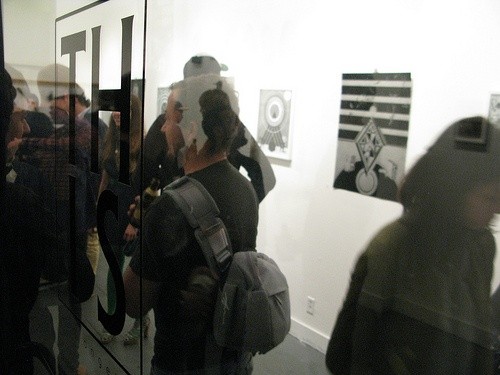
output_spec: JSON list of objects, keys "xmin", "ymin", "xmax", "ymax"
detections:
[{"xmin": 307, "ymin": 297, "xmax": 315, "ymax": 315}]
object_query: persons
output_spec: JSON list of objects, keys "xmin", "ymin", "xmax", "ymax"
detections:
[
  {"xmin": 0, "ymin": 56, "xmax": 277, "ymax": 375},
  {"xmin": 324, "ymin": 116, "xmax": 500, "ymax": 375}
]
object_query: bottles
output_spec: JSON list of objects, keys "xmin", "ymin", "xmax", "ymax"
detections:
[{"xmin": 130, "ymin": 178, "xmax": 161, "ymax": 228}]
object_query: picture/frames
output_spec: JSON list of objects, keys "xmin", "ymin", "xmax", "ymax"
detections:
[{"xmin": 454, "ymin": 115, "xmax": 491, "ymax": 144}]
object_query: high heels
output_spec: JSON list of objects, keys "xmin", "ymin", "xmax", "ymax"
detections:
[
  {"xmin": 121, "ymin": 315, "xmax": 150, "ymax": 345},
  {"xmin": 98, "ymin": 327, "xmax": 114, "ymax": 344}
]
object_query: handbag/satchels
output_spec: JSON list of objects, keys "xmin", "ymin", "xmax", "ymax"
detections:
[{"xmin": 163, "ymin": 175, "xmax": 290, "ymax": 354}]
